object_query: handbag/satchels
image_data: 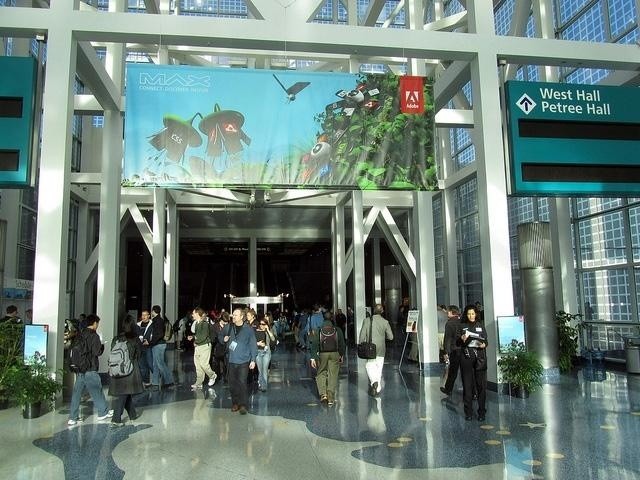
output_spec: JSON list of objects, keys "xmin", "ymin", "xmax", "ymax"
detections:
[
  {"xmin": 215, "ymin": 343, "xmax": 225, "ymax": 358},
  {"xmin": 201, "ymin": 320, "xmax": 217, "ymax": 342},
  {"xmin": 308, "ymin": 330, "xmax": 315, "ymax": 342},
  {"xmin": 164, "ymin": 320, "xmax": 173, "ymax": 341},
  {"xmin": 358, "ymin": 342, "xmax": 376, "ymax": 359}
]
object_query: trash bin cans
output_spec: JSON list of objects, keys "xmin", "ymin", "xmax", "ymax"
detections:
[{"xmin": 625, "ymin": 344, "xmax": 640, "ymax": 374}]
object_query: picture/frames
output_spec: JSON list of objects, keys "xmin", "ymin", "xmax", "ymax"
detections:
[
  {"xmin": 23, "ymin": 324, "xmax": 49, "ymax": 368},
  {"xmin": 496, "ymin": 315, "xmax": 528, "ymax": 354}
]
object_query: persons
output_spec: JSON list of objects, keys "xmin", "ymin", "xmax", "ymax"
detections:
[
  {"xmin": 1, "ymin": 305, "xmax": 24, "ymax": 324},
  {"xmin": 66, "ymin": 314, "xmax": 114, "ymax": 426},
  {"xmin": 397, "ymin": 304, "xmax": 405, "ymax": 322},
  {"xmin": 358, "ymin": 303, "xmax": 394, "ymax": 397},
  {"xmin": 62, "ymin": 313, "xmax": 87, "ymax": 350},
  {"xmin": 439, "ymin": 304, "xmax": 478, "ymax": 400},
  {"xmin": 173, "ymin": 305, "xmax": 346, "ymax": 416},
  {"xmin": 137, "ymin": 310, "xmax": 153, "ymax": 388},
  {"xmin": 107, "ymin": 313, "xmax": 147, "ymax": 427},
  {"xmin": 455, "ymin": 304, "xmax": 488, "ymax": 423},
  {"xmin": 185, "ymin": 387, "xmax": 279, "ymax": 480},
  {"xmin": 142, "ymin": 304, "xmax": 175, "ymax": 392},
  {"xmin": 26, "ymin": 310, "xmax": 33, "ymax": 324}
]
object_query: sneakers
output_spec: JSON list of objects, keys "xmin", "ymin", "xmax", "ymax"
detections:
[
  {"xmin": 370, "ymin": 381, "xmax": 377, "ymax": 397},
  {"xmin": 130, "ymin": 414, "xmax": 141, "ymax": 422},
  {"xmin": 320, "ymin": 394, "xmax": 334, "ymax": 404},
  {"xmin": 97, "ymin": 410, "xmax": 114, "ymax": 421},
  {"xmin": 146, "ymin": 384, "xmax": 158, "ymax": 391},
  {"xmin": 67, "ymin": 417, "xmax": 84, "ymax": 425},
  {"xmin": 190, "ymin": 384, "xmax": 203, "ymax": 389},
  {"xmin": 208, "ymin": 374, "xmax": 217, "ymax": 387},
  {"xmin": 258, "ymin": 383, "xmax": 267, "ymax": 391},
  {"xmin": 108, "ymin": 422, "xmax": 124, "ymax": 428},
  {"xmin": 165, "ymin": 383, "xmax": 176, "ymax": 391}
]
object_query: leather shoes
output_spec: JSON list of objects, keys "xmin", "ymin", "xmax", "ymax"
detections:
[
  {"xmin": 440, "ymin": 386, "xmax": 451, "ymax": 395},
  {"xmin": 466, "ymin": 417, "xmax": 472, "ymax": 420},
  {"xmin": 231, "ymin": 404, "xmax": 239, "ymax": 411},
  {"xmin": 240, "ymin": 406, "xmax": 247, "ymax": 414},
  {"xmin": 479, "ymin": 417, "xmax": 485, "ymax": 421}
]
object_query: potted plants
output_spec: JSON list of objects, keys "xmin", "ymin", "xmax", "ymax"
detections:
[
  {"xmin": 0, "ymin": 317, "xmax": 24, "ymax": 410},
  {"xmin": 3, "ymin": 351, "xmax": 68, "ymax": 420},
  {"xmin": 495, "ymin": 338, "xmax": 547, "ymax": 400}
]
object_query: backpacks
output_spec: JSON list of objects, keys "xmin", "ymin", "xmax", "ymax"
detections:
[
  {"xmin": 68, "ymin": 337, "xmax": 90, "ymax": 374},
  {"xmin": 108, "ymin": 339, "xmax": 134, "ymax": 378},
  {"xmin": 318, "ymin": 326, "xmax": 339, "ymax": 352}
]
